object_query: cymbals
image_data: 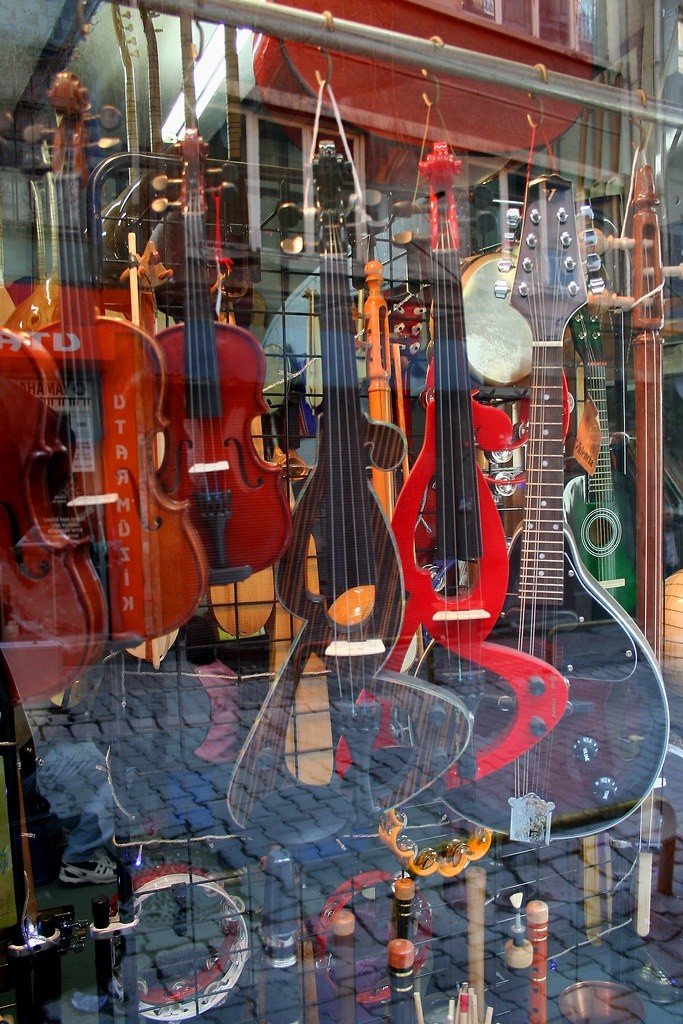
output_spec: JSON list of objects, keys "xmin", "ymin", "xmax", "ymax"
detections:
[
  {"xmin": 310, "ymin": 868, "xmax": 434, "ymax": 1005},
  {"xmin": 99, "ymin": 857, "xmax": 253, "ymax": 1023},
  {"xmin": 377, "ymin": 808, "xmax": 494, "ymax": 878}
]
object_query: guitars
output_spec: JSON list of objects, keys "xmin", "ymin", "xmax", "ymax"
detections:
[
  {"xmin": 226, "ymin": 138, "xmax": 475, "ymax": 848},
  {"xmin": 563, "ymin": 203, "xmax": 640, "ymax": 619},
  {"xmin": 332, "ymin": 136, "xmax": 571, "ymax": 793},
  {"xmin": 432, "ymin": 166, "xmax": 672, "ymax": 843}
]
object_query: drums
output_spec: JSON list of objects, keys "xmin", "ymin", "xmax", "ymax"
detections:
[{"xmin": 457, "ymin": 250, "xmax": 535, "ymax": 385}]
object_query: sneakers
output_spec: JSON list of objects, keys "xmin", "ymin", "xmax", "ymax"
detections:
[{"xmin": 60, "ymin": 852, "xmax": 118, "ymax": 887}]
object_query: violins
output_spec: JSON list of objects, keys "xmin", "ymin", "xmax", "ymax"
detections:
[
  {"xmin": 0, "ymin": 326, "xmax": 111, "ymax": 708},
  {"xmin": 23, "ymin": 70, "xmax": 211, "ymax": 644},
  {"xmin": 150, "ymin": 123, "xmax": 297, "ymax": 588}
]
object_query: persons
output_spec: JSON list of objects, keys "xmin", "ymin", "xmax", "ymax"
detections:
[
  {"xmin": 86, "ymin": 654, "xmax": 168, "ymax": 848},
  {"xmin": 35, "ymin": 698, "xmax": 120, "ymax": 882}
]
